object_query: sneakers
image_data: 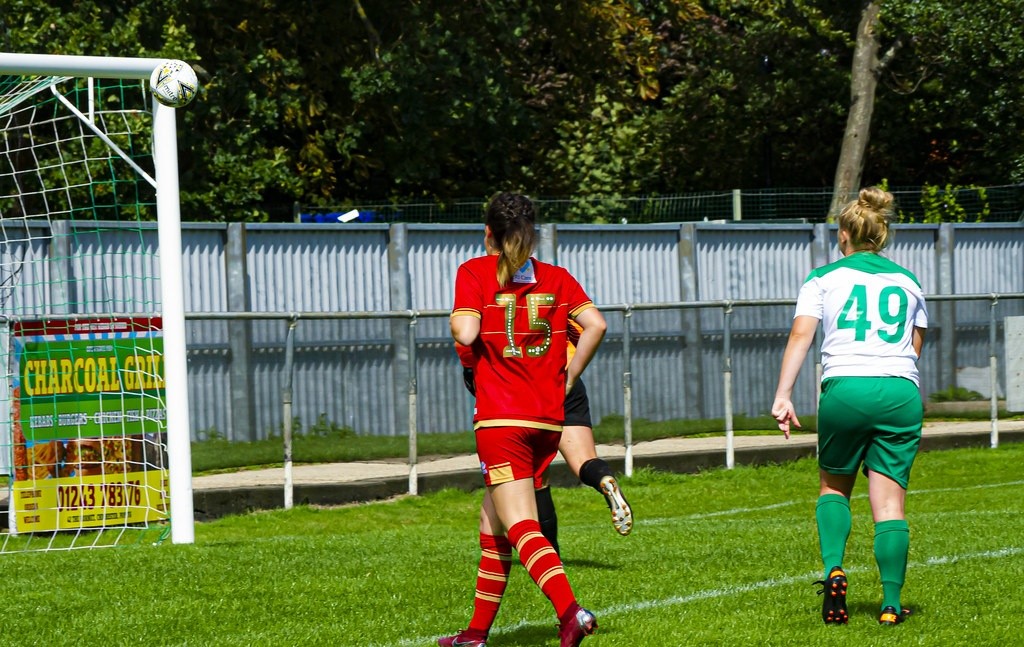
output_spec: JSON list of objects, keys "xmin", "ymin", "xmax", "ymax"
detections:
[
  {"xmin": 597, "ymin": 466, "xmax": 634, "ymax": 537},
  {"xmin": 822, "ymin": 565, "xmax": 849, "ymax": 626},
  {"xmin": 437, "ymin": 632, "xmax": 487, "ymax": 647},
  {"xmin": 559, "ymin": 606, "xmax": 599, "ymax": 647},
  {"xmin": 878, "ymin": 605, "xmax": 906, "ymax": 628}
]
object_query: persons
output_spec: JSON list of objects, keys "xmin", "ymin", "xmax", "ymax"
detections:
[
  {"xmin": 768, "ymin": 189, "xmax": 929, "ymax": 628},
  {"xmin": 437, "ymin": 191, "xmax": 609, "ymax": 646},
  {"xmin": 535, "ymin": 375, "xmax": 635, "ymax": 556}
]
